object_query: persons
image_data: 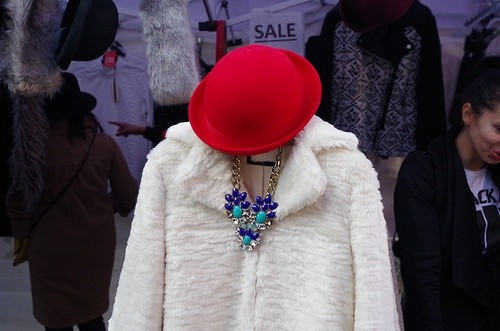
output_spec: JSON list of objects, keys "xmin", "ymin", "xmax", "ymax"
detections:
[
  {"xmin": 108, "ymin": 57, "xmax": 217, "ymax": 148},
  {"xmin": 393, "ymin": 56, "xmax": 500, "ymax": 330},
  {"xmin": 6, "ymin": 72, "xmax": 138, "ymax": 331}
]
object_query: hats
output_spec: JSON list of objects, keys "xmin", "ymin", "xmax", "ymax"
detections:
[
  {"xmin": 41, "ymin": 71, "xmax": 97, "ymax": 120},
  {"xmin": 338, "ymin": 0, "xmax": 415, "ymax": 33},
  {"xmin": 54, "ymin": 0, "xmax": 120, "ymax": 72},
  {"xmin": 188, "ymin": 43, "xmax": 322, "ymax": 157}
]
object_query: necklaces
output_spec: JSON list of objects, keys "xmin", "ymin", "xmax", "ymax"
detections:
[{"xmin": 225, "ymin": 147, "xmax": 283, "ymax": 252}]
